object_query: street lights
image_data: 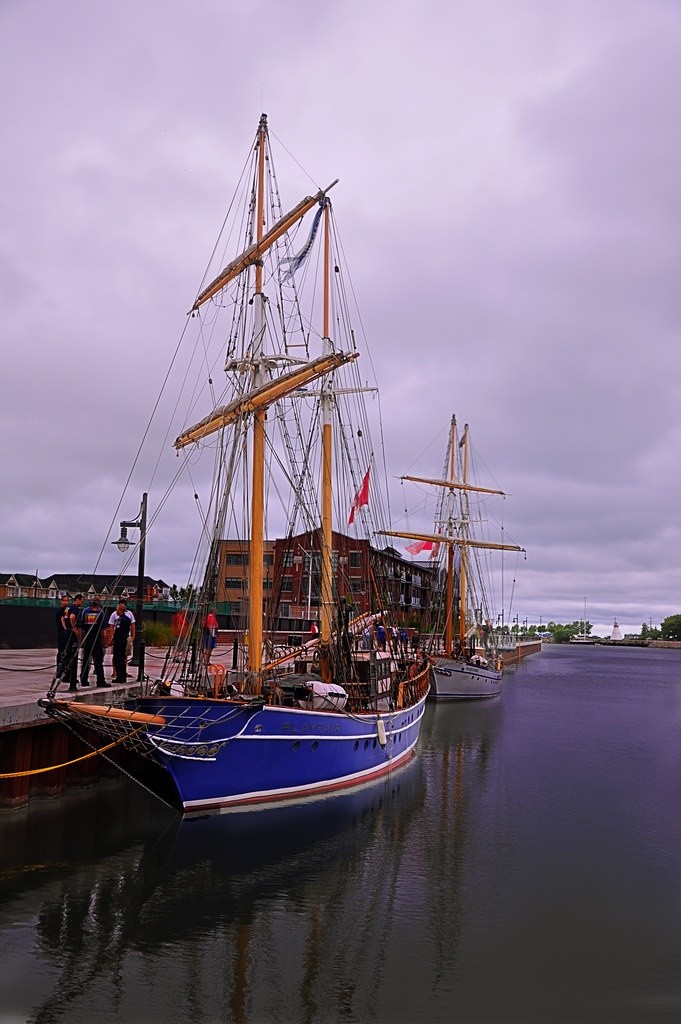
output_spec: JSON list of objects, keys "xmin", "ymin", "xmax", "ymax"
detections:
[
  {"xmin": 111, "ymin": 492, "xmax": 148, "ymax": 683},
  {"xmin": 498, "ymin": 609, "xmax": 527, "ymax": 643}
]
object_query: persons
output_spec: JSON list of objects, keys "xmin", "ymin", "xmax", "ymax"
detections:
[
  {"xmin": 56, "ymin": 595, "xmax": 71, "ymax": 678},
  {"xmin": 111, "ymin": 605, "xmax": 131, "ymax": 682},
  {"xmin": 411, "ymin": 631, "xmax": 420, "ymax": 653},
  {"xmin": 109, "ymin": 599, "xmax": 136, "ymax": 678},
  {"xmin": 204, "ymin": 608, "xmax": 219, "ymax": 666},
  {"xmin": 392, "ymin": 624, "xmax": 398, "ymax": 640},
  {"xmin": 311, "ymin": 622, "xmax": 318, "ymax": 639},
  {"xmin": 362, "ymin": 625, "xmax": 374, "ymax": 650},
  {"xmin": 478, "ymin": 620, "xmax": 492, "ymax": 651},
  {"xmin": 173, "ymin": 605, "xmax": 192, "ymax": 662},
  {"xmin": 377, "ymin": 625, "xmax": 387, "ymax": 646},
  {"xmin": 62, "ymin": 594, "xmax": 84, "ymax": 683},
  {"xmin": 81, "ymin": 595, "xmax": 111, "ymax": 687},
  {"xmin": 400, "ymin": 630, "xmax": 408, "ymax": 651}
]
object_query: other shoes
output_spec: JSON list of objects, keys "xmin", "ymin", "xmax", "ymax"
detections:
[
  {"xmin": 127, "ymin": 673, "xmax": 133, "ymax": 677},
  {"xmin": 173, "ymin": 659, "xmax": 180, "ymax": 663},
  {"xmin": 112, "ymin": 678, "xmax": 126, "ymax": 683},
  {"xmin": 98, "ymin": 683, "xmax": 111, "ymax": 687},
  {"xmin": 111, "ymin": 674, "xmax": 117, "ymax": 677},
  {"xmin": 81, "ymin": 682, "xmax": 90, "ymax": 686},
  {"xmin": 69, "ymin": 687, "xmax": 79, "ymax": 692},
  {"xmin": 206, "ymin": 663, "xmax": 212, "ymax": 666},
  {"xmin": 182, "ymin": 659, "xmax": 189, "ymax": 663}
]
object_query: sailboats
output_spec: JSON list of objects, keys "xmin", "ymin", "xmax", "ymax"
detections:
[
  {"xmin": 39, "ymin": 113, "xmax": 431, "ymax": 817},
  {"xmin": 427, "ymin": 413, "xmax": 504, "ymax": 701}
]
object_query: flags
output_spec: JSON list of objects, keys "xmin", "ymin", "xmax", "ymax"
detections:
[{"xmin": 459, "ymin": 434, "xmax": 466, "ymax": 448}]
